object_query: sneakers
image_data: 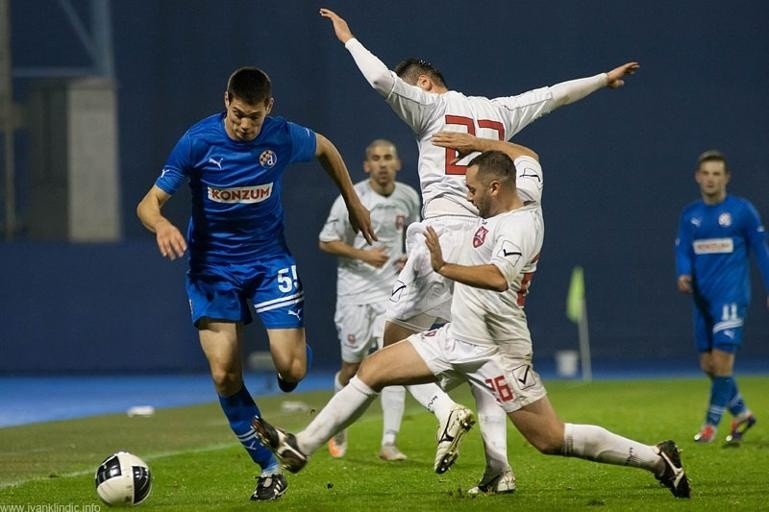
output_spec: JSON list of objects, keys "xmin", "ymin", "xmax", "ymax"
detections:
[
  {"xmin": 435, "ymin": 405, "xmax": 475, "ymax": 473},
  {"xmin": 328, "ymin": 430, "xmax": 347, "ymax": 457},
  {"xmin": 378, "ymin": 445, "xmax": 406, "ymax": 460},
  {"xmin": 468, "ymin": 469, "xmax": 516, "ymax": 494},
  {"xmin": 251, "ymin": 416, "xmax": 306, "ymax": 501},
  {"xmin": 655, "ymin": 440, "xmax": 689, "ymax": 498},
  {"xmin": 695, "ymin": 413, "xmax": 755, "ymax": 443}
]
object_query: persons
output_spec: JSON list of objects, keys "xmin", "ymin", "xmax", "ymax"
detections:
[
  {"xmin": 253, "ymin": 132, "xmax": 692, "ymax": 497},
  {"xmin": 674, "ymin": 151, "xmax": 768, "ymax": 448},
  {"xmin": 319, "ymin": 140, "xmax": 422, "ymax": 462},
  {"xmin": 320, "ymin": 6, "xmax": 642, "ymax": 473},
  {"xmin": 137, "ymin": 67, "xmax": 376, "ymax": 501}
]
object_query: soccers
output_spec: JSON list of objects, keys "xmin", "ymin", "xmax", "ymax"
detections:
[{"xmin": 95, "ymin": 452, "xmax": 153, "ymax": 506}]
